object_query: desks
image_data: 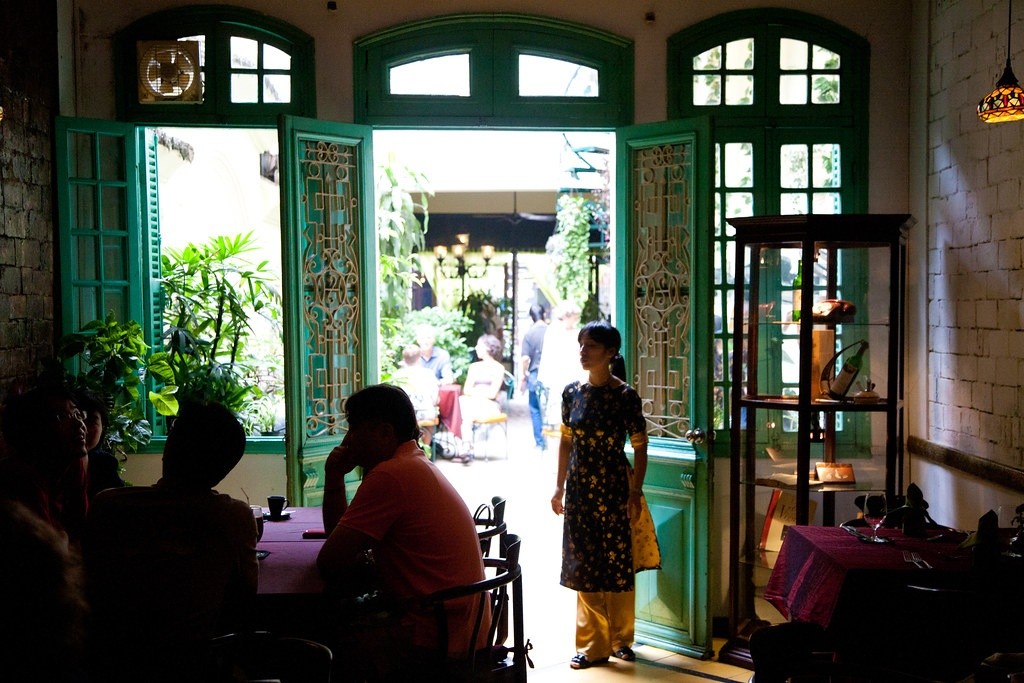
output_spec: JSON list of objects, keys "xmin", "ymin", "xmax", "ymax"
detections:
[
  {"xmin": 764, "ymin": 527, "xmax": 1024, "ymax": 683},
  {"xmin": 438, "ymin": 384, "xmax": 463, "ymax": 439},
  {"xmin": 257, "ymin": 506, "xmax": 371, "ymax": 683}
]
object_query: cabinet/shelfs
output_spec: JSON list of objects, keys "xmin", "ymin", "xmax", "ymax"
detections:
[{"xmin": 718, "ymin": 214, "xmax": 914, "ymax": 671}]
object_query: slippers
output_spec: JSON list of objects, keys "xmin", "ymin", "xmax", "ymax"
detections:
[
  {"xmin": 571, "ymin": 655, "xmax": 609, "ymax": 668},
  {"xmin": 613, "ymin": 647, "xmax": 635, "ymax": 661}
]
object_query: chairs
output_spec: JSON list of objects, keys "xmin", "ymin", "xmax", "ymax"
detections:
[
  {"xmin": 198, "ymin": 499, "xmax": 527, "ymax": 683},
  {"xmin": 416, "ymin": 372, "xmax": 514, "ymax": 463}
]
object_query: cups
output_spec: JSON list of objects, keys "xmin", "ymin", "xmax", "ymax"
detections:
[
  {"xmin": 249, "ymin": 505, "xmax": 264, "ymax": 544},
  {"xmin": 267, "ymin": 496, "xmax": 290, "ymax": 515}
]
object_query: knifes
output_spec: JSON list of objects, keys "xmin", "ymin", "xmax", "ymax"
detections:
[
  {"xmin": 847, "ymin": 526, "xmax": 870, "ymax": 539},
  {"xmin": 840, "ymin": 523, "xmax": 862, "ymax": 538}
]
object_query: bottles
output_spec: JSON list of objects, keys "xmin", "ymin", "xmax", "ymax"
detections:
[
  {"xmin": 829, "ymin": 341, "xmax": 869, "ymax": 402},
  {"xmin": 792, "ymin": 260, "xmax": 802, "ymax": 321}
]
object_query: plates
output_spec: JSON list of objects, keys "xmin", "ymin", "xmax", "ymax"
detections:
[{"xmin": 265, "ymin": 513, "xmax": 290, "ymax": 521}]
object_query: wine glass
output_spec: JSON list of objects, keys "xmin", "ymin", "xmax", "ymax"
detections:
[{"xmin": 863, "ymin": 492, "xmax": 887, "ymax": 543}]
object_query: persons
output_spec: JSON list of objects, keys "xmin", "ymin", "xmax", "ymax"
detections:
[
  {"xmin": 415, "ymin": 324, "xmax": 456, "ymax": 385},
  {"xmin": 551, "ymin": 320, "xmax": 663, "ymax": 669},
  {"xmin": 394, "ymin": 344, "xmax": 440, "ymax": 441},
  {"xmin": 316, "ymin": 383, "xmax": 492, "ymax": 683},
  {"xmin": 459, "ymin": 335, "xmax": 505, "ymax": 463},
  {"xmin": 0, "ymin": 373, "xmax": 124, "ymax": 683},
  {"xmin": 81, "ymin": 400, "xmax": 259, "ymax": 683},
  {"xmin": 520, "ymin": 301, "xmax": 582, "ymax": 451}
]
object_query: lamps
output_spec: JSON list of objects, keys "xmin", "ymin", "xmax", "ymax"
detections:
[{"xmin": 977, "ymin": 0, "xmax": 1024, "ymax": 124}]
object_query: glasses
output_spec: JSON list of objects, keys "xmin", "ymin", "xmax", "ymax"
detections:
[
  {"xmin": 57, "ymin": 410, "xmax": 88, "ymax": 423},
  {"xmin": 166, "ymin": 419, "xmax": 177, "ymax": 438}
]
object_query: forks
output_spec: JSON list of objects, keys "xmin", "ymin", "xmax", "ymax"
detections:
[
  {"xmin": 902, "ymin": 549, "xmax": 923, "ymax": 569},
  {"xmin": 911, "ymin": 552, "xmax": 933, "ymax": 569}
]
